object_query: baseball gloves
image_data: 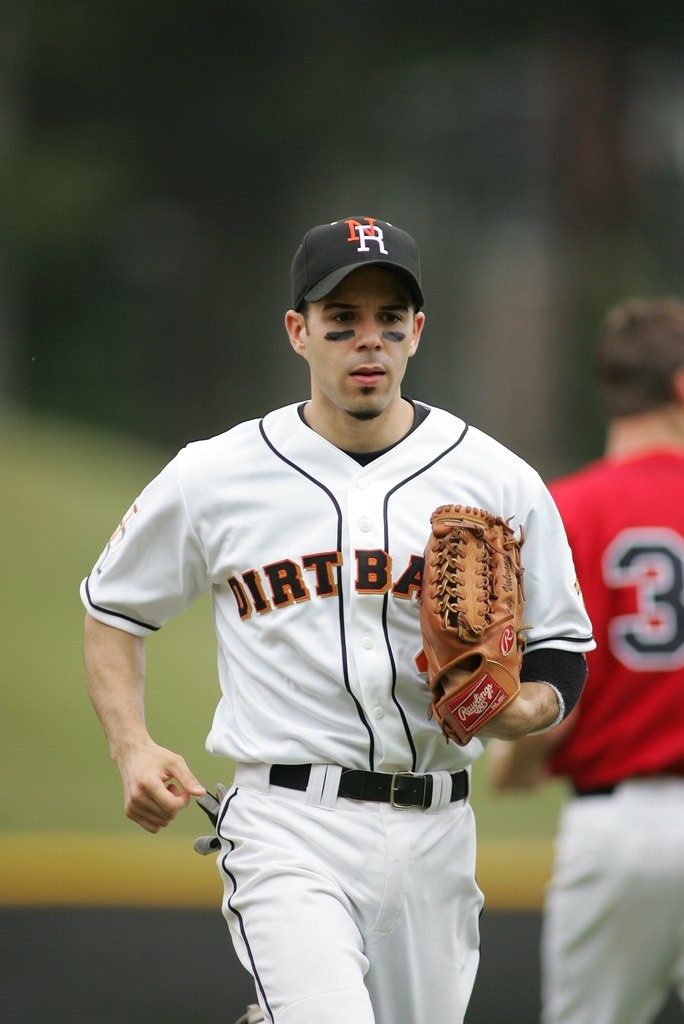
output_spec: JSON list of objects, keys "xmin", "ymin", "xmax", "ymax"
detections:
[{"xmin": 413, "ymin": 502, "xmax": 527, "ymax": 749}]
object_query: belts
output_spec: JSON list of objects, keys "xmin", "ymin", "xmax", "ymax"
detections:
[
  {"xmin": 268, "ymin": 763, "xmax": 468, "ymax": 809},
  {"xmin": 575, "ymin": 760, "xmax": 684, "ymax": 796}
]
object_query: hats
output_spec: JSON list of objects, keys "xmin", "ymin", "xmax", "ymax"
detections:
[{"xmin": 290, "ymin": 217, "xmax": 425, "ymax": 310}]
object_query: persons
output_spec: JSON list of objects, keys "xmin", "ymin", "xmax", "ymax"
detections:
[
  {"xmin": 486, "ymin": 295, "xmax": 684, "ymax": 1024},
  {"xmin": 76, "ymin": 216, "xmax": 595, "ymax": 1024}
]
